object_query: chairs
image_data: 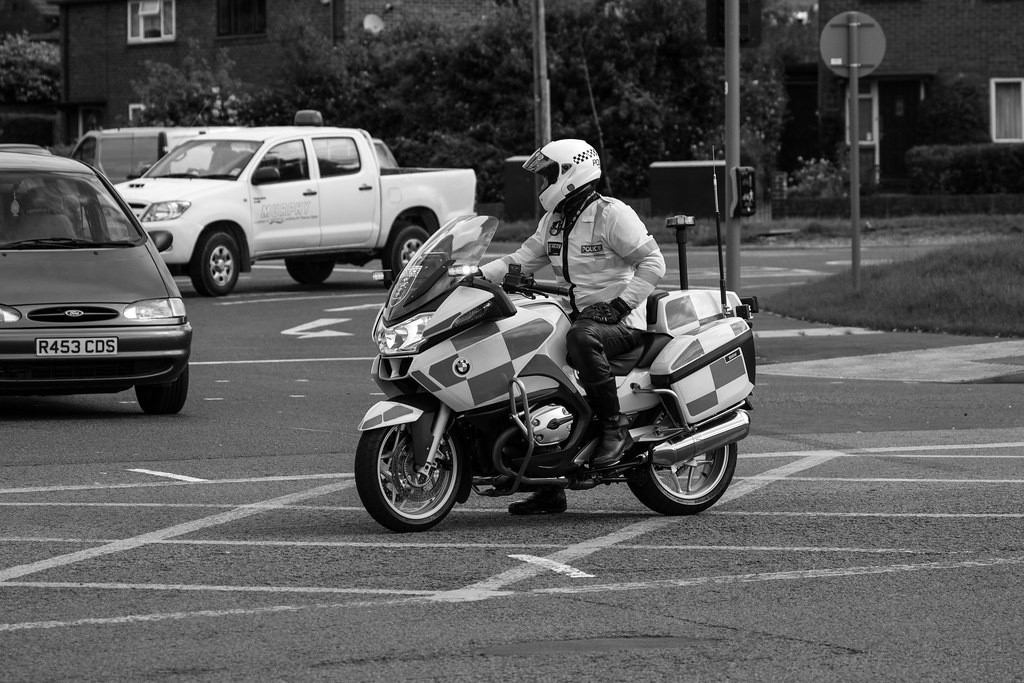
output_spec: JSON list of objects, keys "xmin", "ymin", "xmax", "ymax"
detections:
[{"xmin": 14, "ymin": 186, "xmax": 78, "ymax": 249}]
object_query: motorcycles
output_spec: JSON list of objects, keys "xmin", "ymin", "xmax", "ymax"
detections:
[{"xmin": 354, "ymin": 142, "xmax": 759, "ymax": 534}]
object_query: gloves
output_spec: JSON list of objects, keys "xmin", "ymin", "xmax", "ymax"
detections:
[{"xmin": 578, "ymin": 296, "xmax": 631, "ymax": 324}]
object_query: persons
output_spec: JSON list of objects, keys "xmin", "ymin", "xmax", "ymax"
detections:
[
  {"xmin": 461, "ymin": 139, "xmax": 668, "ymax": 517},
  {"xmin": 186, "ymin": 141, "xmax": 235, "ymax": 178}
]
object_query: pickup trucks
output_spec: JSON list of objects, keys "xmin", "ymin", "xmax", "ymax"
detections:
[{"xmin": 111, "ymin": 109, "xmax": 478, "ymax": 298}]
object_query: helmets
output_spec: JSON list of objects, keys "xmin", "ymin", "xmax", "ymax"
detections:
[{"xmin": 522, "ymin": 138, "xmax": 601, "ymax": 215}]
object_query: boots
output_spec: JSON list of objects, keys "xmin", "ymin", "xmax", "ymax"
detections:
[
  {"xmin": 507, "ymin": 487, "xmax": 566, "ymax": 514},
  {"xmin": 590, "ymin": 377, "xmax": 633, "ymax": 466}
]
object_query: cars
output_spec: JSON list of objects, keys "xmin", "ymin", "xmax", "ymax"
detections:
[{"xmin": 66, "ymin": 128, "xmax": 188, "ymax": 187}]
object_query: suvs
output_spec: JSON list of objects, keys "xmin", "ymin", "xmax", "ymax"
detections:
[{"xmin": 0, "ymin": 144, "xmax": 193, "ymax": 418}]
